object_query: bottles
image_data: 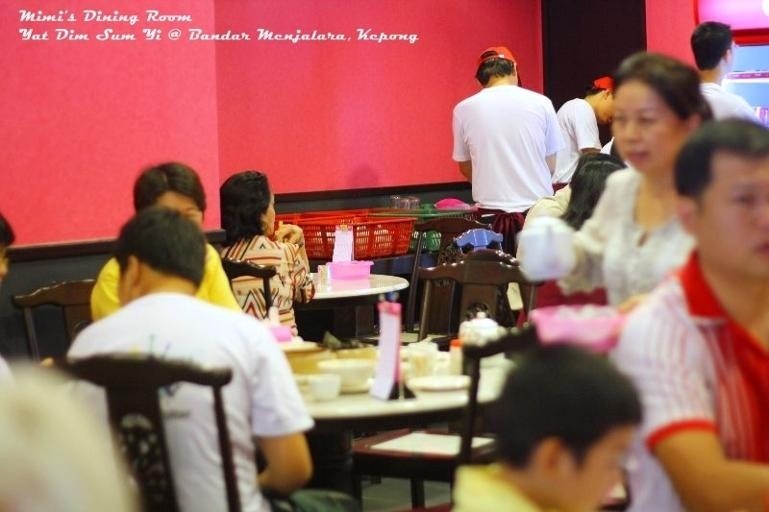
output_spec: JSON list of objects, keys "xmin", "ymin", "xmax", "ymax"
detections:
[
  {"xmin": 450, "ymin": 311, "xmax": 505, "ymax": 399},
  {"xmin": 388, "ymin": 194, "xmax": 421, "ymax": 211}
]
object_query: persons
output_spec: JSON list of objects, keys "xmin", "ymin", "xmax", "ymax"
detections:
[
  {"xmin": 1, "ymin": 356, "xmax": 146, "ymax": 510},
  {"xmin": 213, "ymin": 169, "xmax": 316, "ymax": 341},
  {"xmin": 534, "ymin": 157, "xmax": 626, "ymax": 308},
  {"xmin": 550, "ymin": 76, "xmax": 616, "ymax": 193},
  {"xmin": 87, "ymin": 162, "xmax": 246, "ymax": 320},
  {"xmin": 517, "ymin": 53, "xmax": 719, "ymax": 309},
  {"xmin": 689, "ymin": 21, "xmax": 760, "ymax": 121},
  {"xmin": 607, "ymin": 117, "xmax": 769, "ymax": 509},
  {"xmin": 451, "ymin": 340, "xmax": 639, "ymax": 509},
  {"xmin": 0, "ymin": 213, "xmax": 16, "ymax": 288},
  {"xmin": 68, "ymin": 208, "xmax": 359, "ymax": 509},
  {"xmin": 450, "ymin": 46, "xmax": 564, "ymax": 249}
]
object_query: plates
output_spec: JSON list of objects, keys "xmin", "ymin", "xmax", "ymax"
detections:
[{"xmin": 410, "ymin": 373, "xmax": 478, "ymax": 391}]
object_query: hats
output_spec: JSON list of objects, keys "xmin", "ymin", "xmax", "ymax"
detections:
[
  {"xmin": 476, "ymin": 46, "xmax": 523, "ymax": 87},
  {"xmin": 590, "ymin": 75, "xmax": 613, "ymax": 93}
]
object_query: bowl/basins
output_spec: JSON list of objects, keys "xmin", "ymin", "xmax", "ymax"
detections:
[
  {"xmin": 321, "ymin": 359, "xmax": 370, "ymax": 396},
  {"xmin": 530, "ymin": 305, "xmax": 617, "ymax": 354}
]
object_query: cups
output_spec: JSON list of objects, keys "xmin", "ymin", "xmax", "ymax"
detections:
[
  {"xmin": 308, "ymin": 375, "xmax": 341, "ymax": 400},
  {"xmin": 409, "ymin": 340, "xmax": 437, "ymax": 379}
]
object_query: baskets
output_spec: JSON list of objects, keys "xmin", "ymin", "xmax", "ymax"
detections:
[{"xmin": 269, "ymin": 205, "xmax": 472, "ymax": 259}]
